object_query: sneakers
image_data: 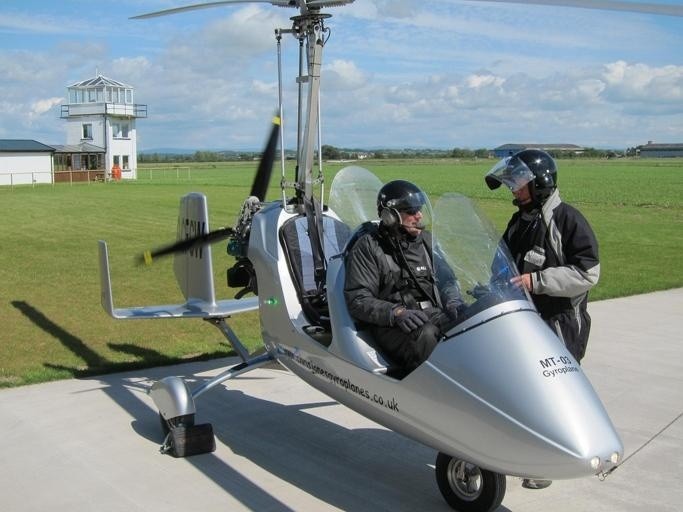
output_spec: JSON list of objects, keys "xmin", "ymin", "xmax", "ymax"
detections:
[{"xmin": 524, "ymin": 478, "xmax": 552, "ymax": 489}]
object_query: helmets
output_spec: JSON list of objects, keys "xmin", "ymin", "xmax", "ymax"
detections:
[
  {"xmin": 484, "ymin": 149, "xmax": 557, "ymax": 206},
  {"xmin": 377, "ymin": 180, "xmax": 426, "ymax": 231}
]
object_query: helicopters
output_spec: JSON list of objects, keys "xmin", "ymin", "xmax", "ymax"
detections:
[{"xmin": 96, "ymin": 0, "xmax": 683, "ymax": 512}]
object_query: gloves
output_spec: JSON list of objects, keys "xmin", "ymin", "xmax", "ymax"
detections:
[
  {"xmin": 391, "ymin": 306, "xmax": 428, "ymax": 334},
  {"xmin": 446, "ymin": 299, "xmax": 469, "ymax": 319}
]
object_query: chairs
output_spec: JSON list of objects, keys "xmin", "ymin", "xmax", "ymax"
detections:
[{"xmin": 279, "ymin": 213, "xmax": 398, "ymax": 374}]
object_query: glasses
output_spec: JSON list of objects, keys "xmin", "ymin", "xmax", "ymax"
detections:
[{"xmin": 399, "ymin": 208, "xmax": 421, "ymax": 215}]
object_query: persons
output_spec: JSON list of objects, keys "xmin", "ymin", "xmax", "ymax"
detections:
[
  {"xmin": 483, "ymin": 150, "xmax": 598, "ymax": 369},
  {"xmin": 342, "ymin": 179, "xmax": 469, "ymax": 378}
]
